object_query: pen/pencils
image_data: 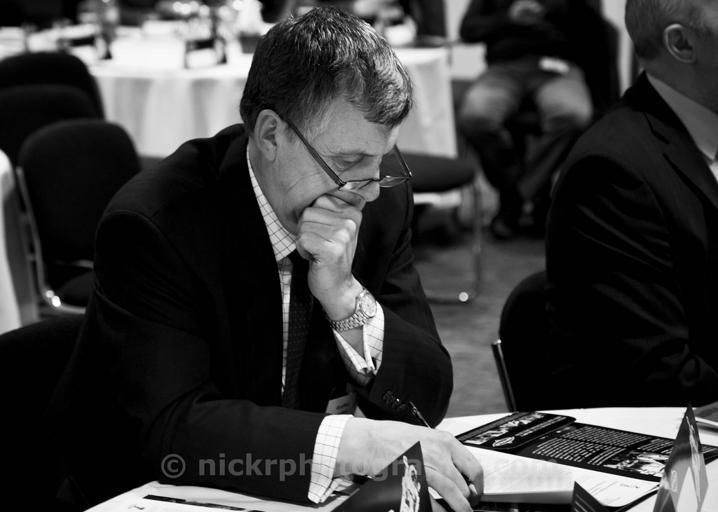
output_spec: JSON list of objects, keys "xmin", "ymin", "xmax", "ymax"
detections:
[{"xmin": 407, "ymin": 400, "xmax": 479, "ymax": 497}]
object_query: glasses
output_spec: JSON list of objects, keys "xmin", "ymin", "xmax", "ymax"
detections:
[{"xmin": 284, "ymin": 117, "xmax": 413, "ymax": 193}]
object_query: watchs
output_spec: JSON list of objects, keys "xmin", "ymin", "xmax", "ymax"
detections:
[{"xmin": 326, "ymin": 285, "xmax": 378, "ymax": 332}]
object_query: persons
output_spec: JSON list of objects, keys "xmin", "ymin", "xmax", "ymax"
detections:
[
  {"xmin": 38, "ymin": 6, "xmax": 485, "ymax": 512},
  {"xmin": 538, "ymin": 1, "xmax": 716, "ymax": 404},
  {"xmin": 457, "ymin": 0, "xmax": 597, "ymax": 242}
]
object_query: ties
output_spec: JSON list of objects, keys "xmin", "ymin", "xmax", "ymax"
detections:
[{"xmin": 284, "ymin": 247, "xmax": 320, "ymax": 412}]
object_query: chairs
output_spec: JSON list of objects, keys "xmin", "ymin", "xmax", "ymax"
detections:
[
  {"xmin": 1, "ymin": 315, "xmax": 88, "ymax": 512},
  {"xmin": 488, "ymin": 270, "xmax": 612, "ymax": 412},
  {"xmin": 1, "ymin": 0, "xmax": 637, "ymax": 323}
]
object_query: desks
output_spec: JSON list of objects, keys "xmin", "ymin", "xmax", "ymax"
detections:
[{"xmin": 80, "ymin": 405, "xmax": 718, "ymax": 512}]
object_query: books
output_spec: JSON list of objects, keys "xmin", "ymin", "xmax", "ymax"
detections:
[
  {"xmin": 330, "ymin": 439, "xmax": 660, "ymax": 512},
  {"xmin": 453, "ymin": 409, "xmax": 717, "ymax": 483}
]
